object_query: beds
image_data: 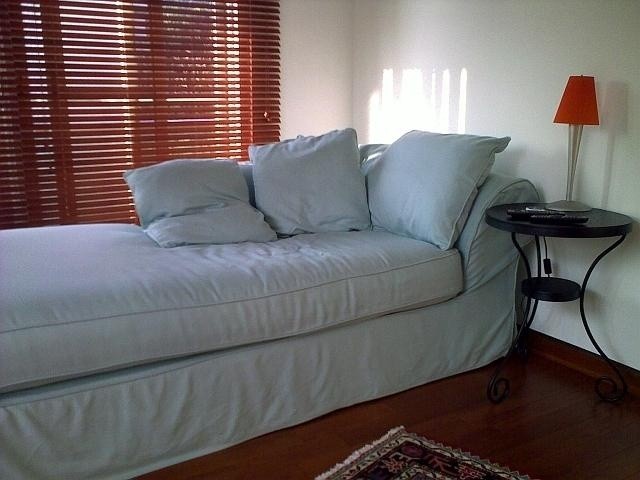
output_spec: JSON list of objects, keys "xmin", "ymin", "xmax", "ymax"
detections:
[{"xmin": 1, "ymin": 141, "xmax": 542, "ymax": 480}]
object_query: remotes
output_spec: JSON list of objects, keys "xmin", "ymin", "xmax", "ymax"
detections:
[
  {"xmin": 531, "ymin": 215, "xmax": 589, "ymax": 223},
  {"xmin": 526, "ymin": 207, "xmax": 546, "ymax": 212},
  {"xmin": 507, "ymin": 209, "xmax": 566, "ymax": 217}
]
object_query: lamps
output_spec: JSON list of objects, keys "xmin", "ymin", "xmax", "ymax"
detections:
[{"xmin": 546, "ymin": 76, "xmax": 600, "ymax": 213}]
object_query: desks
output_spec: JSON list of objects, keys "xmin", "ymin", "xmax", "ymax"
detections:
[{"xmin": 485, "ymin": 203, "xmax": 632, "ymax": 405}]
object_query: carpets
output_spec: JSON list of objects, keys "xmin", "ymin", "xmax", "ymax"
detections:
[{"xmin": 314, "ymin": 425, "xmax": 533, "ymax": 479}]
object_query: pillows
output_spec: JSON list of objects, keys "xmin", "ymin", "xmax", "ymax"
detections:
[
  {"xmin": 123, "ymin": 159, "xmax": 277, "ymax": 249},
  {"xmin": 248, "ymin": 128, "xmax": 371, "ymax": 239},
  {"xmin": 366, "ymin": 129, "xmax": 511, "ymax": 252}
]
